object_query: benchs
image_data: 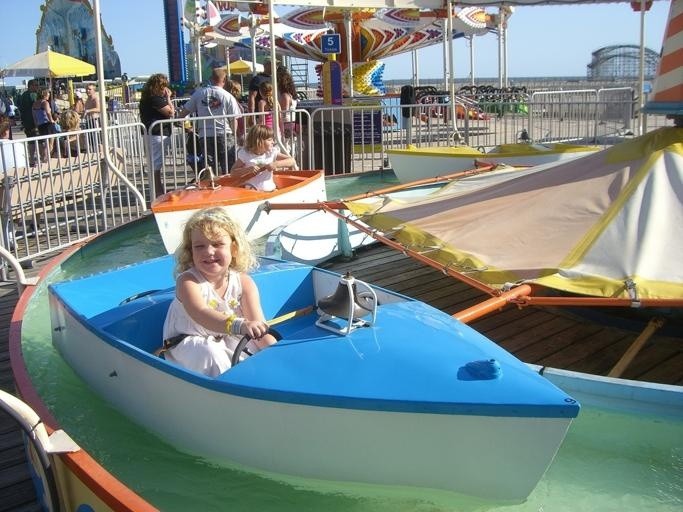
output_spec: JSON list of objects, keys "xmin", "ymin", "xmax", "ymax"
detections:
[{"xmin": 0, "ymin": 144, "xmax": 127, "ymax": 277}]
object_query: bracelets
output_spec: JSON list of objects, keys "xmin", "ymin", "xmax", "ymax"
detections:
[
  {"xmin": 224, "ymin": 313, "xmax": 237, "ymax": 336},
  {"xmin": 230, "ymin": 315, "xmax": 249, "ymax": 336}
]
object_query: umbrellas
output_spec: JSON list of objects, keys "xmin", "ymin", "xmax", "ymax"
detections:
[
  {"xmin": 213, "ymin": 55, "xmax": 265, "ymax": 97},
  {"xmin": 0, "ymin": 45, "xmax": 97, "ymax": 114}
]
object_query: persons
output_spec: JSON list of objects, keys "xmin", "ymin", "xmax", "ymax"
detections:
[
  {"xmin": 137, "ymin": 74, "xmax": 176, "ymax": 200},
  {"xmin": 223, "ymin": 80, "xmax": 247, "ymax": 150},
  {"xmin": 230, "ymin": 124, "xmax": 297, "ymax": 195},
  {"xmin": 257, "ymin": 80, "xmax": 283, "ymax": 138},
  {"xmin": 0, "ymin": 78, "xmax": 117, "ymax": 168},
  {"xmin": 0, "ymin": 112, "xmax": 41, "ymax": 253},
  {"xmin": 172, "ymin": 67, "xmax": 243, "ymax": 181},
  {"xmin": 246, "ymin": 55, "xmax": 282, "ymax": 128},
  {"xmin": 277, "ymin": 65, "xmax": 298, "ymax": 171},
  {"xmin": 159, "ymin": 205, "xmax": 279, "ymax": 380}
]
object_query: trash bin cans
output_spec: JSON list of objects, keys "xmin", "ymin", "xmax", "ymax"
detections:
[
  {"xmin": 0, "ymin": 86, "xmax": 17, "ymax": 115},
  {"xmin": 108, "ymin": 99, "xmax": 118, "ymax": 111},
  {"xmin": 296, "ymin": 101, "xmax": 323, "ymax": 168}
]
